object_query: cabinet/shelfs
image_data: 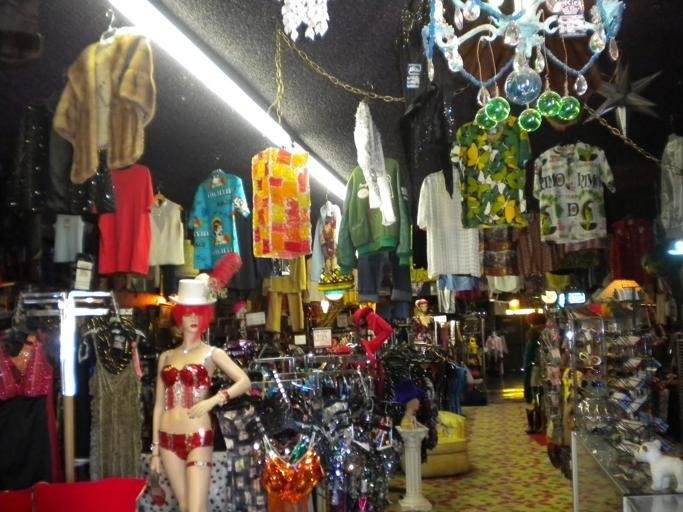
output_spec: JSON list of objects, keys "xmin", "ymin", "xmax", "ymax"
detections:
[
  {"xmin": 571, "ymin": 306, "xmax": 659, "ymax": 434},
  {"xmin": 462, "ymin": 317, "xmax": 486, "ymax": 379},
  {"xmin": 570, "ymin": 428, "xmax": 683, "ymax": 512}
]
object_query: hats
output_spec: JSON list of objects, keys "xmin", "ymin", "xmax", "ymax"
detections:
[
  {"xmin": 528, "ymin": 313, "xmax": 544, "ymax": 324},
  {"xmin": 394, "ymin": 382, "xmax": 426, "ymax": 404},
  {"xmin": 168, "ymin": 279, "xmax": 217, "ymax": 306}
]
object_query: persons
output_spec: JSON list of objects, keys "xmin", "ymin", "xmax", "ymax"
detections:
[
  {"xmin": 523, "ymin": 313, "xmax": 546, "ymax": 434},
  {"xmin": 414, "ymin": 299, "xmax": 433, "ymax": 326},
  {"xmin": 149, "ymin": 279, "xmax": 252, "ymax": 511}
]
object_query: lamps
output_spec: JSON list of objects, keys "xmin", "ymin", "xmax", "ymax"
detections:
[{"xmin": 422, "ymin": 1, "xmax": 627, "ymax": 134}]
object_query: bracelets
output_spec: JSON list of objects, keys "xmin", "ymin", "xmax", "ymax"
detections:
[
  {"xmin": 151, "ymin": 443, "xmax": 159, "ymax": 451},
  {"xmin": 222, "ymin": 390, "xmax": 231, "ymax": 404},
  {"xmin": 216, "ymin": 391, "xmax": 225, "ymax": 407}
]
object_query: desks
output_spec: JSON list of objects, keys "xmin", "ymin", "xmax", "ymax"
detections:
[
  {"xmin": 0, "ymin": 477, "xmax": 150, "ymax": 511},
  {"xmin": 623, "ymin": 492, "xmax": 683, "ymax": 512}
]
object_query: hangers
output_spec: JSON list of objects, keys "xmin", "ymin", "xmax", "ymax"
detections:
[
  {"xmin": 266, "ymin": 402, "xmax": 302, "ymax": 438},
  {"xmin": 83, "ymin": 316, "xmax": 146, "ymax": 338},
  {"xmin": 559, "ymin": 136, "xmax": 578, "ymax": 148},
  {"xmin": 152, "ymin": 183, "xmax": 166, "ymax": 205},
  {"xmin": 97, "ymin": 8, "xmax": 119, "ymax": 46}
]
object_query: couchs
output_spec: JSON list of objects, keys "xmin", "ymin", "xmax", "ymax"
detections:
[{"xmin": 399, "ymin": 410, "xmax": 468, "ymax": 479}]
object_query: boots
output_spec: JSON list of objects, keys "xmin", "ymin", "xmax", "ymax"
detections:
[
  {"xmin": 534, "ymin": 405, "xmax": 541, "ymax": 433},
  {"xmin": 526, "ymin": 409, "xmax": 534, "ymax": 433}
]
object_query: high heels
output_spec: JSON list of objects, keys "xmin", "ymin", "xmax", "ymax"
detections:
[{"xmin": 150, "ymin": 473, "xmax": 165, "ymax": 506}]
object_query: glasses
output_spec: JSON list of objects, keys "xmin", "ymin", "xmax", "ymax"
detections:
[
  {"xmin": 576, "ymin": 340, "xmax": 591, "ymax": 349},
  {"xmin": 579, "ymin": 352, "xmax": 601, "ymax": 365},
  {"xmin": 562, "ymin": 327, "xmax": 576, "ymax": 431},
  {"xmin": 582, "ymin": 380, "xmax": 600, "ymax": 388},
  {"xmin": 580, "ymin": 368, "xmax": 600, "ymax": 377}
]
